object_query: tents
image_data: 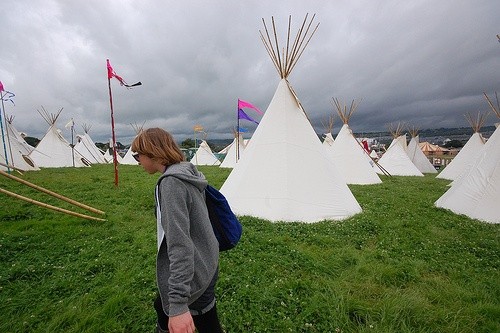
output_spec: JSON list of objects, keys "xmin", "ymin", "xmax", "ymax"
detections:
[
  {"xmin": 215, "ymin": 79, "xmax": 362, "ymax": 224},
  {"xmin": 27, "ymin": 125, "xmax": 91, "ymax": 167},
  {"xmin": 5, "ymin": 124, "xmax": 34, "ymax": 155},
  {"xmin": 328, "ymin": 123, "xmax": 382, "ymax": 184},
  {"xmin": 104, "ymin": 148, "xmax": 122, "ymax": 163},
  {"xmin": 73, "ymin": 134, "xmax": 111, "ymax": 164},
  {"xmin": 119, "ymin": 147, "xmax": 140, "ymax": 165},
  {"xmin": 190, "ymin": 140, "xmax": 221, "ymax": 166},
  {"xmin": 372, "ymin": 138, "xmax": 424, "ymax": 176},
  {"xmin": 436, "ymin": 132, "xmax": 485, "ymax": 180},
  {"xmin": 434, "ymin": 125, "xmax": 500, "ymax": 223},
  {"xmin": 0, "ymin": 128, "xmax": 41, "ymax": 171},
  {"xmin": 218, "ymin": 139, "xmax": 245, "ymax": 168},
  {"xmin": 322, "ymin": 132, "xmax": 334, "ymax": 149},
  {"xmin": 407, "ymin": 137, "xmax": 438, "ymax": 173},
  {"xmin": 419, "ymin": 142, "xmax": 441, "ymax": 156}
]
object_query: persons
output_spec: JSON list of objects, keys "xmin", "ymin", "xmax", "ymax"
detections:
[{"xmin": 131, "ymin": 127, "xmax": 224, "ymax": 333}]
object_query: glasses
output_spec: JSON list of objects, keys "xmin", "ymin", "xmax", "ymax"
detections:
[{"xmin": 132, "ymin": 153, "xmax": 141, "ymax": 162}]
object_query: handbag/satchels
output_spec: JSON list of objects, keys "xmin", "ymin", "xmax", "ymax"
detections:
[{"xmin": 204, "ymin": 181, "xmax": 242, "ymax": 251}]
